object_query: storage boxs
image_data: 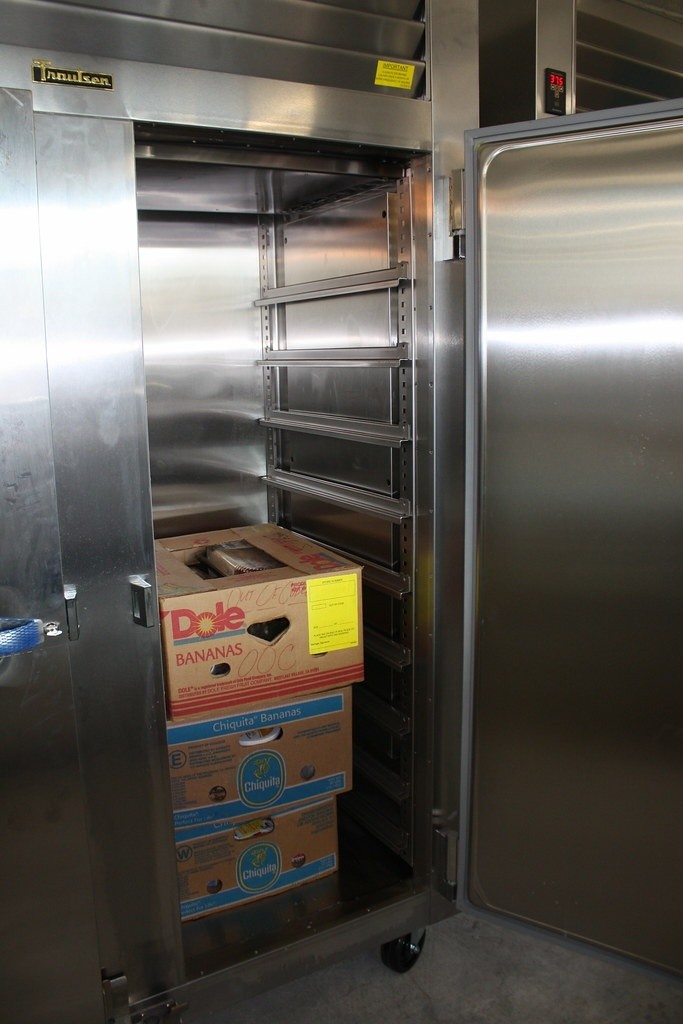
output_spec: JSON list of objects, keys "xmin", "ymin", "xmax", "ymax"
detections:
[
  {"xmin": 175, "ymin": 798, "xmax": 338, "ymax": 924},
  {"xmin": 153, "ymin": 522, "xmax": 366, "ymax": 720},
  {"xmin": 165, "ymin": 687, "xmax": 352, "ymax": 833}
]
